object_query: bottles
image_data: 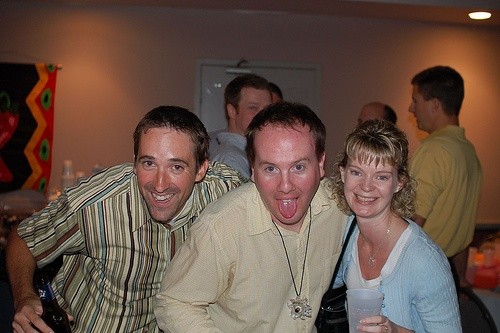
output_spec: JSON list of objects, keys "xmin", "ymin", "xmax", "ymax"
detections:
[{"xmin": 35, "ymin": 269, "xmax": 72, "ymax": 333}]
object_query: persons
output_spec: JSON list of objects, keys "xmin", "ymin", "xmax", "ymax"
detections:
[
  {"xmin": 357, "ymin": 101, "xmax": 398, "ymax": 135},
  {"xmin": 313, "ymin": 131, "xmax": 462, "ymax": 333},
  {"xmin": 6, "ymin": 105, "xmax": 250, "ymax": 333},
  {"xmin": 266, "ymin": 81, "xmax": 284, "ymax": 105},
  {"xmin": 154, "ymin": 100, "xmax": 354, "ymax": 333},
  {"xmin": 407, "ymin": 64, "xmax": 483, "ymax": 304},
  {"xmin": 200, "ymin": 72, "xmax": 274, "ymax": 180}
]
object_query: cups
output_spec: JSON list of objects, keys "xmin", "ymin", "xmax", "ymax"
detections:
[
  {"xmin": 62, "ymin": 159, "xmax": 75, "ymax": 189},
  {"xmin": 346, "ymin": 288, "xmax": 384, "ymax": 333}
]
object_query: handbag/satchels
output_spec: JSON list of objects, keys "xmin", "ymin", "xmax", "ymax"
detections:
[{"xmin": 314, "ymin": 283, "xmax": 349, "ymax": 333}]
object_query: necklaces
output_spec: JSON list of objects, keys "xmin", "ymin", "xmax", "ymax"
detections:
[
  {"xmin": 361, "ymin": 216, "xmax": 392, "ymax": 269},
  {"xmin": 273, "ymin": 205, "xmax": 313, "ymax": 321}
]
